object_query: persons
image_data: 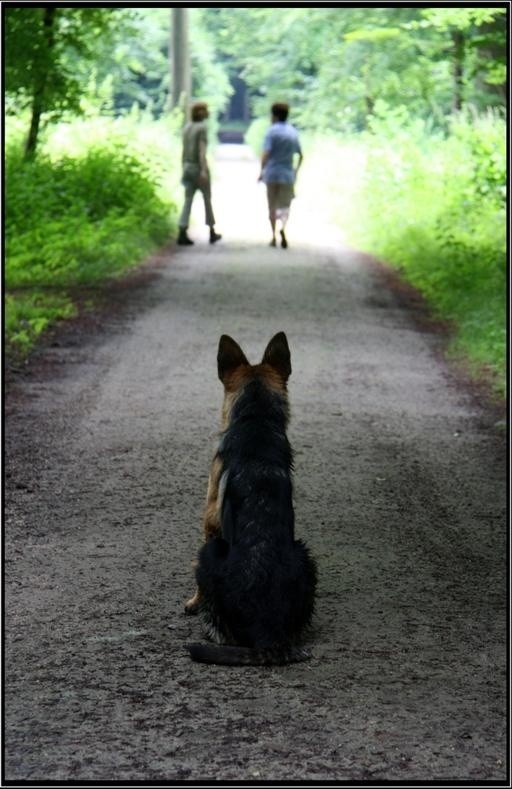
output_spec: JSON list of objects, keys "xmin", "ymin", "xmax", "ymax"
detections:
[
  {"xmin": 258, "ymin": 103, "xmax": 304, "ymax": 249},
  {"xmin": 176, "ymin": 101, "xmax": 223, "ymax": 245}
]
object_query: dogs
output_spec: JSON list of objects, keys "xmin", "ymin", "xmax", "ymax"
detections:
[{"xmin": 181, "ymin": 331, "xmax": 320, "ymax": 667}]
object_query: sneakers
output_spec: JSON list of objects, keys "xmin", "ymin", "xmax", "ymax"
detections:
[{"xmin": 177, "ymin": 235, "xmax": 221, "ymax": 244}]
